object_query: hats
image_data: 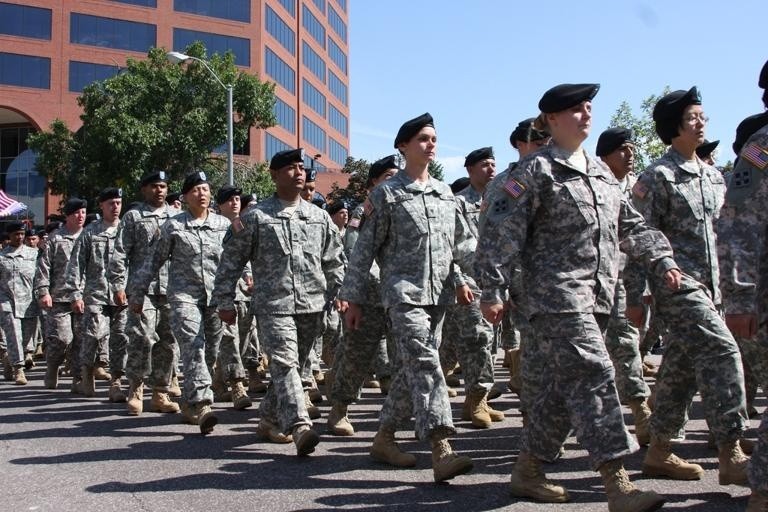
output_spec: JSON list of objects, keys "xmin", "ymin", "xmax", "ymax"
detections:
[
  {"xmin": 595, "ymin": 127, "xmax": 633, "ymax": 158},
  {"xmin": 305, "ymin": 170, "xmax": 348, "ymax": 217},
  {"xmin": 463, "ymin": 146, "xmax": 495, "ymax": 167},
  {"xmin": 269, "ymin": 148, "xmax": 306, "ymax": 170},
  {"xmin": 1, "ymin": 165, "xmax": 257, "ymax": 236},
  {"xmin": 696, "ymin": 138, "xmax": 721, "ymax": 159},
  {"xmin": 654, "ymin": 85, "xmax": 703, "ymax": 110},
  {"xmin": 510, "ymin": 117, "xmax": 553, "ymax": 149},
  {"xmin": 365, "ymin": 154, "xmax": 401, "ymax": 189},
  {"xmin": 538, "ymin": 82, "xmax": 601, "ymax": 113},
  {"xmin": 395, "ymin": 113, "xmax": 434, "ymax": 149},
  {"xmin": 733, "ymin": 110, "xmax": 768, "ymax": 157}
]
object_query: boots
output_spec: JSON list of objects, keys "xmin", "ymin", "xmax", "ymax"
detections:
[
  {"xmin": 257, "ymin": 354, "xmax": 766, "ymax": 512},
  {"xmin": 2, "ymin": 349, "xmax": 268, "ymax": 435}
]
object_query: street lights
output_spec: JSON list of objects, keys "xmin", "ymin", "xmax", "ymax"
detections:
[{"xmin": 166, "ymin": 49, "xmax": 235, "ymax": 187}]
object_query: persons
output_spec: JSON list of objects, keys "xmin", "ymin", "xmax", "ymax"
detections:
[
  {"xmin": 326, "ymin": 64, "xmax": 767, "ymax": 512},
  {"xmin": 1, "ymin": 149, "xmax": 347, "ymax": 457}
]
